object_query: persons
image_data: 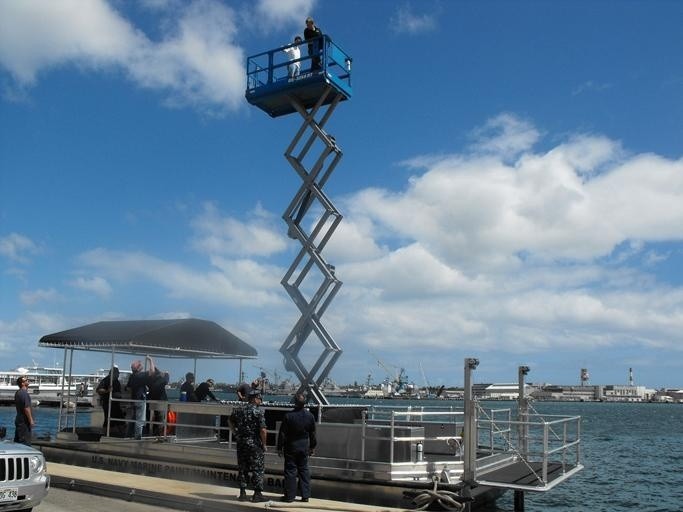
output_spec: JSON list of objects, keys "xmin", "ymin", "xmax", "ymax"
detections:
[
  {"xmin": 124, "ymin": 356, "xmax": 154, "ymax": 439},
  {"xmin": 181, "ymin": 372, "xmax": 196, "ymax": 400},
  {"xmin": 147, "ymin": 367, "xmax": 169, "ymax": 436},
  {"xmin": 304, "ymin": 18, "xmax": 323, "ymax": 71},
  {"xmin": 281, "ymin": 36, "xmax": 303, "ymax": 77},
  {"xmin": 80, "ymin": 383, "xmax": 84, "ymax": 397},
  {"xmin": 277, "ymin": 395, "xmax": 317, "ymax": 502},
  {"xmin": 195, "ymin": 378, "xmax": 219, "ymax": 401},
  {"xmin": 14, "ymin": 376, "xmax": 34, "ymax": 447},
  {"xmin": 227, "ymin": 390, "xmax": 271, "ymax": 503},
  {"xmin": 96, "ymin": 367, "xmax": 126, "ymax": 438},
  {"xmin": 237, "ymin": 379, "xmax": 260, "ymax": 402}
]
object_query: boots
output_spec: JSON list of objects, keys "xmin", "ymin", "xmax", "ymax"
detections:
[
  {"xmin": 252, "ymin": 489, "xmax": 269, "ymax": 502},
  {"xmin": 238, "ymin": 490, "xmax": 247, "ymax": 501}
]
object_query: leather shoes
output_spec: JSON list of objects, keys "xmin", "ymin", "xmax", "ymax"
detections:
[
  {"xmin": 301, "ymin": 497, "xmax": 309, "ymax": 502},
  {"xmin": 280, "ymin": 496, "xmax": 293, "ymax": 501}
]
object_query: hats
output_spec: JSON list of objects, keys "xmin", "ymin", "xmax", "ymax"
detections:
[
  {"xmin": 131, "ymin": 360, "xmax": 140, "ymax": 373},
  {"xmin": 249, "ymin": 389, "xmax": 262, "ymax": 399}
]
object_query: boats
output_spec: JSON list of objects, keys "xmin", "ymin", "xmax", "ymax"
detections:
[
  {"xmin": 0, "ymin": 366, "xmax": 133, "ymax": 407},
  {"xmin": 32, "ymin": 359, "xmax": 585, "ymax": 511}
]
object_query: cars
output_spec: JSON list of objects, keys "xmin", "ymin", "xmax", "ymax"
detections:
[{"xmin": 0, "ymin": 429, "xmax": 49, "ymax": 512}]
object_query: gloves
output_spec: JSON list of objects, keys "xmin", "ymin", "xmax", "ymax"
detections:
[
  {"xmin": 233, "ymin": 432, "xmax": 240, "ymax": 442},
  {"xmin": 261, "ymin": 443, "xmax": 267, "ymax": 452}
]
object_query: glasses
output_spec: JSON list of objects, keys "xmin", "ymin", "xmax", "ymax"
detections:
[{"xmin": 21, "ymin": 379, "xmax": 27, "ymax": 383}]
